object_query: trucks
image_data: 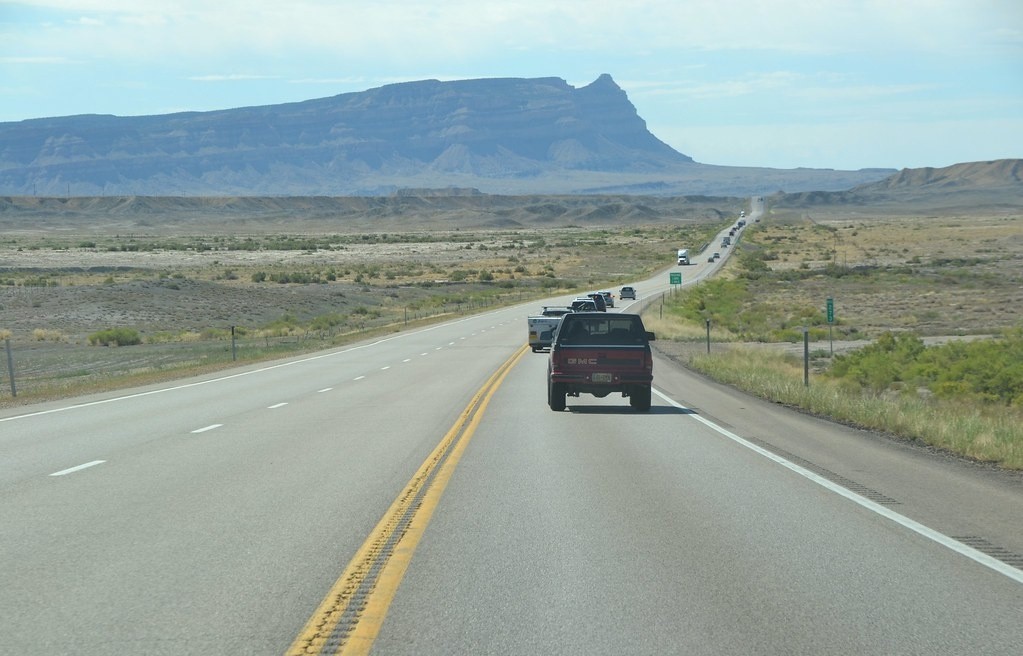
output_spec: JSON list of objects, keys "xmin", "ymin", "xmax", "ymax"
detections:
[
  {"xmin": 678, "ymin": 249, "xmax": 689, "ymax": 265},
  {"xmin": 528, "ymin": 305, "xmax": 576, "ymax": 352}
]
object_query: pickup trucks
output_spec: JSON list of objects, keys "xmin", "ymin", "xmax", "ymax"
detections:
[{"xmin": 546, "ymin": 312, "xmax": 656, "ymax": 412}]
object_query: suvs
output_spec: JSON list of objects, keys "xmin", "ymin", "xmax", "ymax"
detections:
[
  {"xmin": 571, "ymin": 297, "xmax": 598, "ymax": 312},
  {"xmin": 587, "ymin": 294, "xmax": 607, "ymax": 312}
]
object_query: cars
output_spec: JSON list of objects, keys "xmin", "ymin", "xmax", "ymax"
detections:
[
  {"xmin": 597, "ymin": 291, "xmax": 615, "ymax": 308},
  {"xmin": 619, "ymin": 287, "xmax": 637, "ymax": 300},
  {"xmin": 720, "ymin": 218, "xmax": 746, "ymax": 248},
  {"xmin": 713, "ymin": 252, "xmax": 720, "ymax": 258},
  {"xmin": 708, "ymin": 257, "xmax": 714, "ymax": 262}
]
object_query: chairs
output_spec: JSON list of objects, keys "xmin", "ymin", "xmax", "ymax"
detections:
[
  {"xmin": 610, "ymin": 328, "xmax": 628, "ymax": 337},
  {"xmin": 568, "ymin": 329, "xmax": 587, "ymax": 336}
]
